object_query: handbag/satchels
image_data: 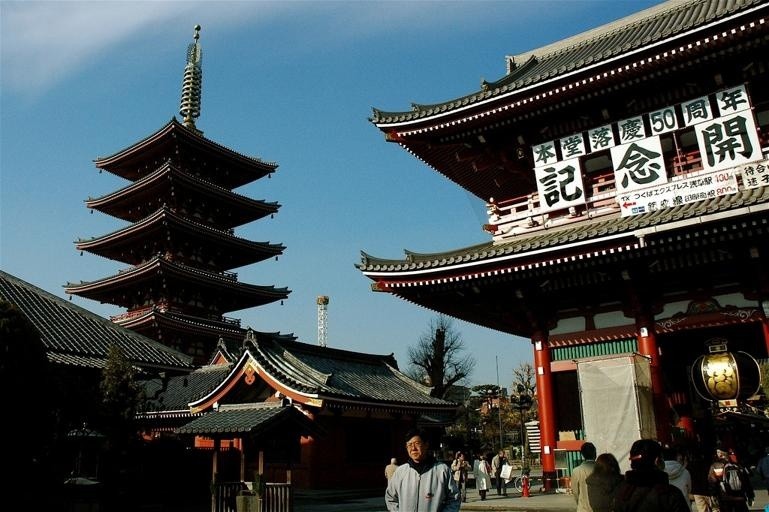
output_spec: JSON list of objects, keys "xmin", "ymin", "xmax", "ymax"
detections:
[{"xmin": 500, "ymin": 465, "xmax": 513, "ymax": 480}]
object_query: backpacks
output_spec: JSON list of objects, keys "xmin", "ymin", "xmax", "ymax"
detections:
[{"xmin": 720, "ymin": 459, "xmax": 744, "ymax": 497}]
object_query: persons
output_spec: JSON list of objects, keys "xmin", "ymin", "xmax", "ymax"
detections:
[
  {"xmin": 450, "ymin": 451, "xmax": 472, "ymax": 503},
  {"xmin": 569, "ymin": 433, "xmax": 769, "ymax": 512},
  {"xmin": 385, "ymin": 456, "xmax": 399, "ymax": 484},
  {"xmin": 473, "ymin": 450, "xmax": 492, "ymax": 501},
  {"xmin": 384, "ymin": 429, "xmax": 462, "ymax": 512},
  {"xmin": 491, "ymin": 449, "xmax": 510, "ymax": 496}
]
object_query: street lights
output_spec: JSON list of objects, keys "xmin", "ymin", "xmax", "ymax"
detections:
[{"xmin": 511, "ymin": 385, "xmax": 532, "ymax": 466}]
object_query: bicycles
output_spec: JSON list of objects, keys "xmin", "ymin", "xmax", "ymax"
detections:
[{"xmin": 515, "ymin": 467, "xmax": 532, "ymax": 493}]
